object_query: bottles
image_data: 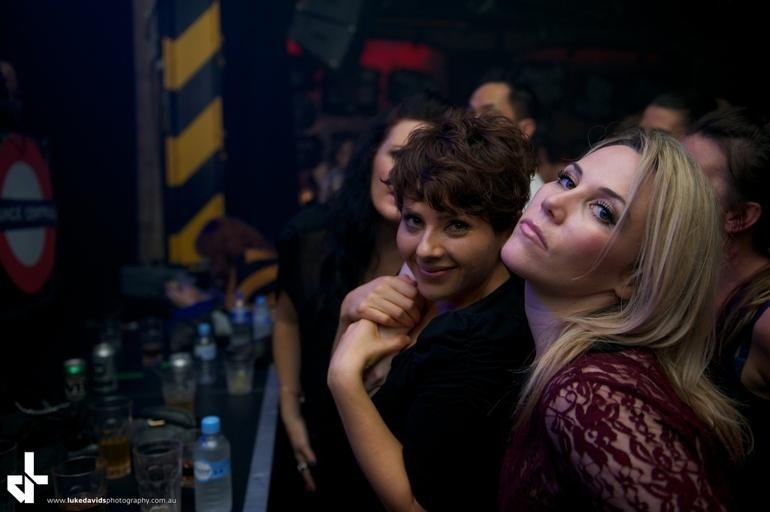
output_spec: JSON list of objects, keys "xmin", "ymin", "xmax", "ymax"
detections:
[
  {"xmin": 252, "ymin": 297, "xmax": 272, "ymax": 365},
  {"xmin": 193, "ymin": 415, "xmax": 233, "ymax": 512},
  {"xmin": 229, "ymin": 291, "xmax": 252, "ymax": 362},
  {"xmin": 194, "ymin": 324, "xmax": 218, "ymax": 386}
]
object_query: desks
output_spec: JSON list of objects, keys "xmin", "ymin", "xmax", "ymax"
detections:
[{"xmin": 0, "ymin": 296, "xmax": 278, "ymax": 512}]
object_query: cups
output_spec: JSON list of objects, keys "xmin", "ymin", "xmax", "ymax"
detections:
[
  {"xmin": 50, "ymin": 456, "xmax": 108, "ymax": 512},
  {"xmin": 85, "ymin": 395, "xmax": 132, "ymax": 479},
  {"xmin": 132, "ymin": 438, "xmax": 184, "ymax": 512},
  {"xmin": 223, "ymin": 349, "xmax": 255, "ymax": 397}
]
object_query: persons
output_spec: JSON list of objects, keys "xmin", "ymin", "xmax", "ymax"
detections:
[{"xmin": 142, "ymin": 62, "xmax": 770, "ymax": 512}]
